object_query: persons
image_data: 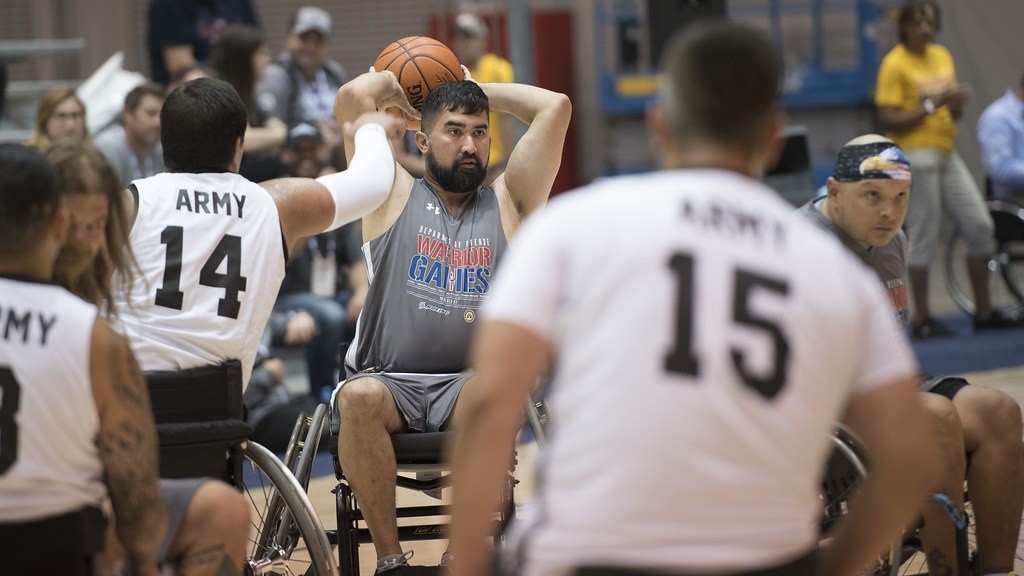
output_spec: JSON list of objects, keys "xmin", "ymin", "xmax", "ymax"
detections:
[
  {"xmin": 205, "ymin": 23, "xmax": 291, "ymax": 183},
  {"xmin": 1, "ymin": 136, "xmax": 166, "ymax": 575},
  {"xmin": 263, "ymin": 122, "xmax": 372, "ymax": 405},
  {"xmin": 788, "ymin": 133, "xmax": 1023, "ymax": 575},
  {"xmin": 433, "ymin": 10, "xmax": 519, "ymax": 172},
  {"xmin": 52, "ymin": 147, "xmax": 252, "ymax": 575},
  {"xmin": 92, "ymin": 86, "xmax": 168, "ymax": 185},
  {"xmin": 166, "ymin": 68, "xmax": 221, "ymax": 94},
  {"xmin": 35, "ymin": 86, "xmax": 90, "ymax": 149},
  {"xmin": 145, "ymin": 2, "xmax": 260, "ymax": 81},
  {"xmin": 252, "ymin": 5, "xmax": 346, "ymax": 122},
  {"xmin": 977, "ymin": 75, "xmax": 1024, "ymax": 205},
  {"xmin": 99, "ymin": 81, "xmax": 413, "ymax": 418},
  {"xmin": 873, "ymin": 0, "xmax": 1023, "ymax": 340},
  {"xmin": 446, "ymin": 20, "xmax": 940, "ymax": 576}
]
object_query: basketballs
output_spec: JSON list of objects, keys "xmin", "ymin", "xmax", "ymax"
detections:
[{"xmin": 373, "ymin": 35, "xmax": 465, "ymax": 132}]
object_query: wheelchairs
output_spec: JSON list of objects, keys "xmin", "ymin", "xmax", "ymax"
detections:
[
  {"xmin": 140, "ymin": 358, "xmax": 342, "ymax": 576},
  {"xmin": 942, "ymin": 168, "xmax": 1023, "ymax": 319},
  {"xmin": 245, "ymin": 367, "xmax": 551, "ymax": 576},
  {"xmin": 824, "ymin": 420, "xmax": 1024, "ymax": 576}
]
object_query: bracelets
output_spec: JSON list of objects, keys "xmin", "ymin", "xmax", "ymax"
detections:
[{"xmin": 918, "ymin": 90, "xmax": 937, "ymax": 116}]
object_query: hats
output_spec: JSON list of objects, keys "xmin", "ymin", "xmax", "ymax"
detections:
[
  {"xmin": 287, "ymin": 5, "xmax": 333, "ymax": 34},
  {"xmin": 451, "ymin": 11, "xmax": 488, "ymax": 36}
]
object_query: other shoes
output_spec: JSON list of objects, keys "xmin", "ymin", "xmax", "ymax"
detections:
[
  {"xmin": 910, "ymin": 317, "xmax": 954, "ymax": 339},
  {"xmin": 970, "ymin": 309, "xmax": 1023, "ymax": 332}
]
